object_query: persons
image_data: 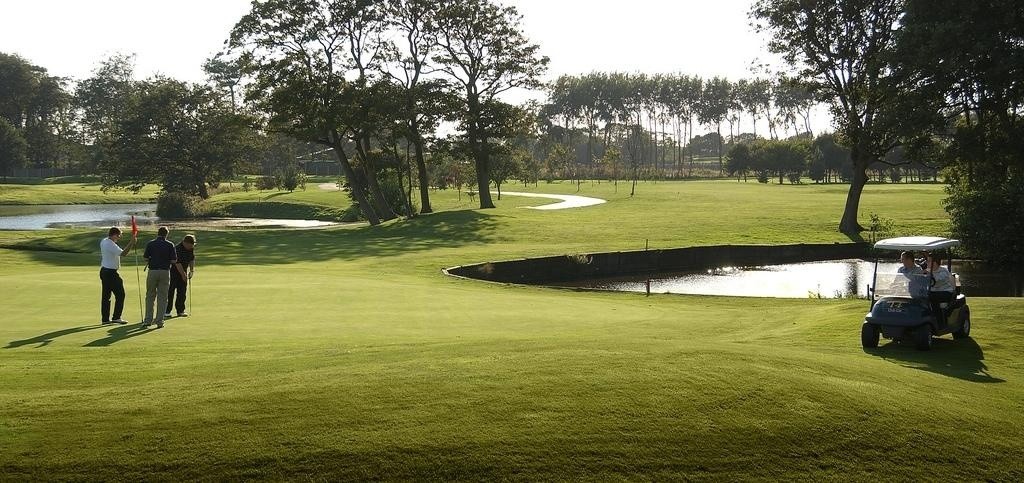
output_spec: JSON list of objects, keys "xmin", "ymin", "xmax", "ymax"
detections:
[
  {"xmin": 142, "ymin": 226, "xmax": 177, "ymax": 326},
  {"xmin": 99, "ymin": 227, "xmax": 138, "ymax": 323},
  {"xmin": 887, "ymin": 251, "xmax": 924, "ymax": 295},
  {"xmin": 165, "ymin": 234, "xmax": 196, "ymax": 316},
  {"xmin": 916, "ymin": 255, "xmax": 956, "ymax": 329}
]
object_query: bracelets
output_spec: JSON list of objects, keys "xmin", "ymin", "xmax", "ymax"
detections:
[{"xmin": 190, "ymin": 270, "xmax": 194, "ymax": 272}]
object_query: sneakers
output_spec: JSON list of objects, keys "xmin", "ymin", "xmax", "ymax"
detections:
[
  {"xmin": 101, "ymin": 317, "xmax": 128, "ymax": 325},
  {"xmin": 165, "ymin": 311, "xmax": 187, "ymax": 317},
  {"xmin": 142, "ymin": 324, "xmax": 164, "ymax": 327}
]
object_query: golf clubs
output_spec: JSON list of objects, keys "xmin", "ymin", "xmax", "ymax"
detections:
[{"xmin": 186, "ymin": 272, "xmax": 192, "ymax": 317}]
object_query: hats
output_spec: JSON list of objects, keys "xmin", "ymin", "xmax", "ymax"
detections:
[{"xmin": 183, "ymin": 234, "xmax": 198, "ymax": 244}]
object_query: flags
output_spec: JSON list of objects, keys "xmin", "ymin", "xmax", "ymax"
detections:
[{"xmin": 132, "ymin": 215, "xmax": 138, "ymax": 236}]
